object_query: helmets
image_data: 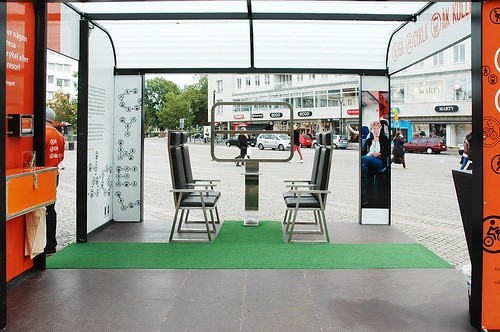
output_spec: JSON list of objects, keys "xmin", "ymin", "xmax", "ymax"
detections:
[{"xmin": 46, "ymin": 108, "xmax": 55, "ymax": 123}]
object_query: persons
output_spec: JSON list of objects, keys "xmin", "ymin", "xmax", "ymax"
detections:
[
  {"xmin": 391, "ymin": 132, "xmax": 406, "ymax": 168},
  {"xmin": 289, "ymin": 127, "xmax": 304, "ymax": 162},
  {"xmin": 234, "ymin": 127, "xmax": 248, "ymax": 165},
  {"xmin": 362, "ymin": 121, "xmax": 389, "ymax": 205},
  {"xmin": 461, "ymin": 132, "xmax": 472, "ymax": 170},
  {"xmin": 44, "ymin": 108, "xmax": 65, "ymax": 254}
]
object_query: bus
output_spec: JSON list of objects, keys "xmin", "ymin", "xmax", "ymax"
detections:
[{"xmin": 52, "ymin": 121, "xmax": 73, "ymax": 142}]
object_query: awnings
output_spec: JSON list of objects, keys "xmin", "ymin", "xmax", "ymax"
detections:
[{"xmin": 246, "ymin": 124, "xmax": 271, "ymax": 130}]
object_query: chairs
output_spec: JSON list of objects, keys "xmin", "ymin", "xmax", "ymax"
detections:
[
  {"xmin": 167, "ymin": 129, "xmax": 222, "ymax": 243},
  {"xmin": 282, "ymin": 129, "xmax": 335, "ymax": 243}
]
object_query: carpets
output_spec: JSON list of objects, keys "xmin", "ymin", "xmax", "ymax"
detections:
[{"xmin": 46, "ymin": 220, "xmax": 456, "ymax": 271}]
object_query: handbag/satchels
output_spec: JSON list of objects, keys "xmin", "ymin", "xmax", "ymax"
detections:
[
  {"xmin": 394, "ymin": 157, "xmax": 402, "ymax": 164},
  {"xmin": 298, "ymin": 129, "xmax": 305, "ymax": 144}
]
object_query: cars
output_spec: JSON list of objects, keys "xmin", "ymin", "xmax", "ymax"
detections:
[
  {"xmin": 225, "ymin": 133, "xmax": 256, "ymax": 147},
  {"xmin": 299, "ymin": 133, "xmax": 312, "ymax": 148},
  {"xmin": 255, "ymin": 133, "xmax": 291, "ymax": 152},
  {"xmin": 402, "ymin": 136, "xmax": 447, "ymax": 154},
  {"xmin": 190, "ymin": 133, "xmax": 203, "ymax": 139},
  {"xmin": 311, "ymin": 134, "xmax": 349, "ymax": 149}
]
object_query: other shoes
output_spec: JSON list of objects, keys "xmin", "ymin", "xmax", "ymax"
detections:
[
  {"xmin": 44, "ymin": 246, "xmax": 56, "ymax": 255},
  {"xmin": 236, "ymin": 164, "xmax": 242, "ymax": 166},
  {"xmin": 298, "ymin": 160, "xmax": 304, "ymax": 162}
]
